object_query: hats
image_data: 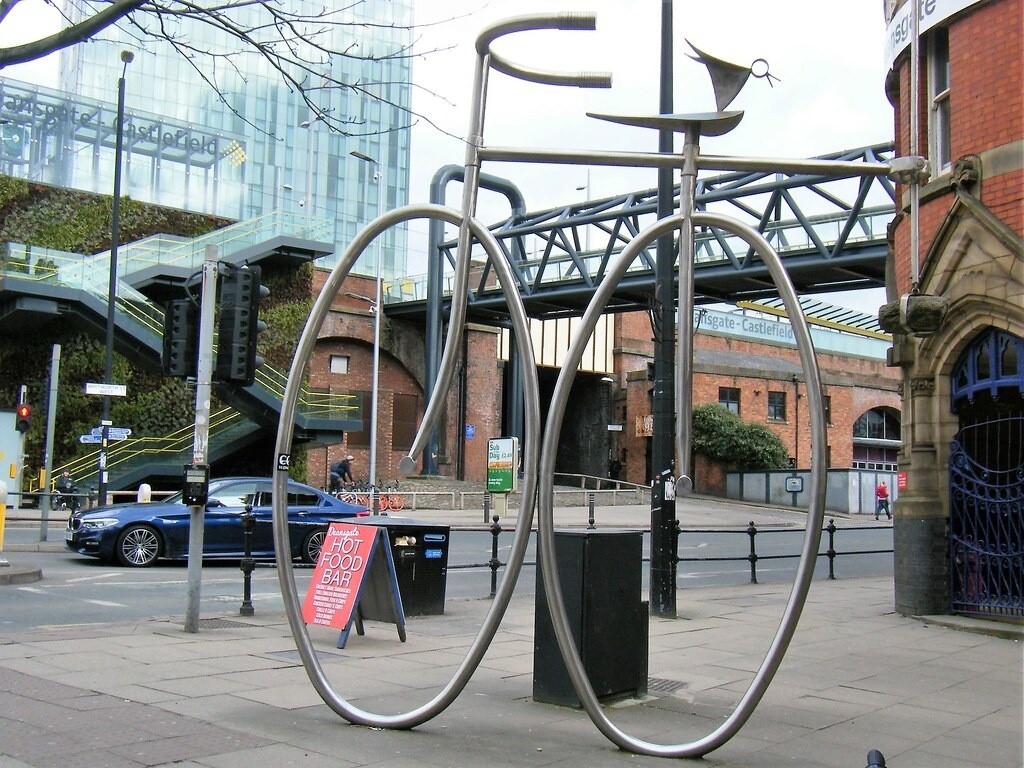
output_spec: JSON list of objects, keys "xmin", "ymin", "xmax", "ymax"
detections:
[
  {"xmin": 346, "ymin": 455, "xmax": 354, "ymax": 461},
  {"xmin": 614, "ymin": 457, "xmax": 618, "ymax": 458}
]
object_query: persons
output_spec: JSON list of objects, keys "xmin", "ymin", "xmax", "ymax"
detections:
[
  {"xmin": 873, "ymin": 481, "xmax": 893, "ymax": 521},
  {"xmin": 327, "ymin": 454, "xmax": 357, "ymax": 500},
  {"xmin": 56, "ymin": 467, "xmax": 81, "ymax": 511}
]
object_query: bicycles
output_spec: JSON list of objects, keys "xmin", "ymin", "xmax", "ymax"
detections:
[
  {"xmin": 268, "ymin": 13, "xmax": 927, "ymax": 754},
  {"xmin": 316, "ymin": 479, "xmax": 407, "ymax": 512},
  {"xmin": 49, "ymin": 486, "xmax": 81, "ymax": 510}
]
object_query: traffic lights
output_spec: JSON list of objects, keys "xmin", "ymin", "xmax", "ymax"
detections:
[
  {"xmin": 217, "ymin": 264, "xmax": 270, "ymax": 387},
  {"xmin": 14, "ymin": 402, "xmax": 34, "ymax": 433}
]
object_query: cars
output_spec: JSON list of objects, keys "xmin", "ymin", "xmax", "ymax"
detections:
[{"xmin": 65, "ymin": 477, "xmax": 377, "ymax": 568}]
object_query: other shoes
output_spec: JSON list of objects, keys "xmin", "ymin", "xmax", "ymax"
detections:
[
  {"xmin": 876, "ymin": 516, "xmax": 878, "ymax": 520},
  {"xmin": 888, "ymin": 516, "xmax": 892, "ymax": 519}
]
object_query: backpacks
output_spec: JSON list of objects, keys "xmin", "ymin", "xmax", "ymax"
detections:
[{"xmin": 330, "ymin": 461, "xmax": 343, "ymax": 472}]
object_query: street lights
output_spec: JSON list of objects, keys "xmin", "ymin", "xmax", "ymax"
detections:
[{"xmin": 95, "ymin": 50, "xmax": 135, "ymax": 509}]
